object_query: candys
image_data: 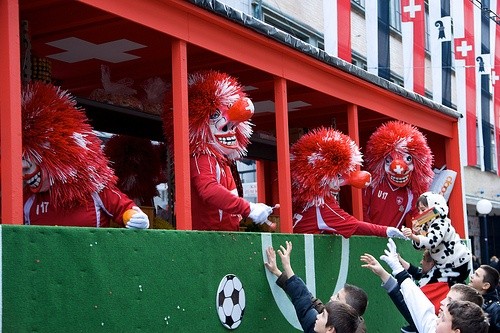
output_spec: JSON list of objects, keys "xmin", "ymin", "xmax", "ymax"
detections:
[
  {"xmin": 272, "ymin": 204, "xmax": 280, "ymax": 209},
  {"xmin": 265, "ymin": 220, "xmax": 277, "ymax": 229}
]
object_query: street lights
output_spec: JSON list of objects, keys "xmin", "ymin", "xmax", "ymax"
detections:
[{"xmin": 475, "ymin": 199, "xmax": 492, "ymax": 265}]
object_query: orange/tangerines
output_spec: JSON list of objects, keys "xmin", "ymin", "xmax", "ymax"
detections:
[{"xmin": 123, "ymin": 209, "xmax": 137, "ymax": 225}]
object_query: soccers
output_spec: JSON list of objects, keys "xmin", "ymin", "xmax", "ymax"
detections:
[{"xmin": 216, "ymin": 273, "xmax": 246, "ymax": 330}]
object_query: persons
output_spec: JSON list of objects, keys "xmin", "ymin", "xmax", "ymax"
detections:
[
  {"xmin": 160, "ymin": 70, "xmax": 275, "ymax": 232},
  {"xmin": 470, "ymin": 265, "xmax": 500, "ymax": 333},
  {"xmin": 20, "ymin": 82, "xmax": 151, "ymax": 229},
  {"xmin": 101, "ymin": 131, "xmax": 163, "ymax": 230},
  {"xmin": 489, "ymin": 256, "xmax": 500, "ymax": 271},
  {"xmin": 362, "ymin": 121, "xmax": 435, "ymax": 234},
  {"xmin": 382, "ymin": 238, "xmax": 490, "ymax": 333},
  {"xmin": 264, "ymin": 246, "xmax": 368, "ymax": 333},
  {"xmin": 394, "ymin": 248, "xmax": 449, "ymax": 317},
  {"xmin": 359, "ymin": 252, "xmax": 484, "ymax": 333},
  {"xmin": 398, "ymin": 191, "xmax": 471, "ymax": 333},
  {"xmin": 289, "ymin": 125, "xmax": 408, "ymax": 241},
  {"xmin": 279, "ymin": 241, "xmax": 358, "ymax": 333}
]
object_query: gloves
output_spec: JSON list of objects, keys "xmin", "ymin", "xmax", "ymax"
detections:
[
  {"xmin": 380, "ymin": 238, "xmax": 404, "ymax": 276},
  {"xmin": 386, "ymin": 227, "xmax": 406, "ymax": 239},
  {"xmin": 125, "ymin": 206, "xmax": 149, "ymax": 230},
  {"xmin": 247, "ymin": 202, "xmax": 273, "ymax": 225}
]
